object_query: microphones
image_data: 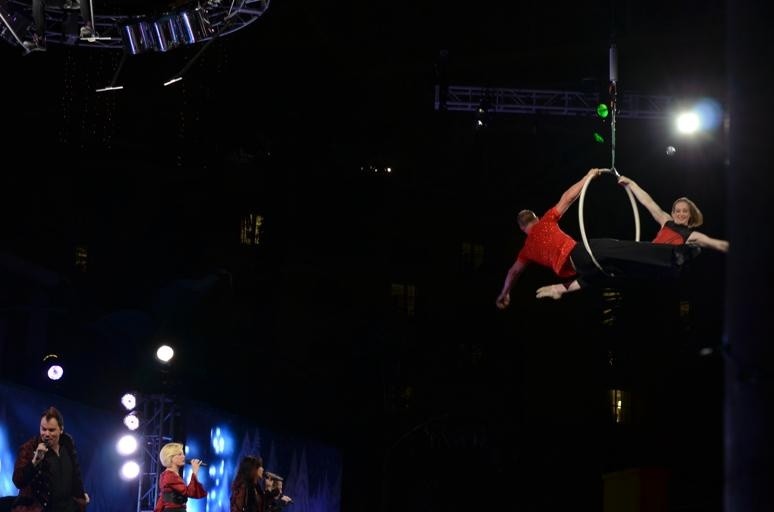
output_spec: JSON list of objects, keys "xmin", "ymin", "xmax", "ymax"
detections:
[
  {"xmin": 185, "ymin": 459, "xmax": 207, "ymax": 466},
  {"xmin": 263, "ymin": 471, "xmax": 284, "ymax": 481},
  {"xmin": 282, "ymin": 495, "xmax": 295, "ymax": 505},
  {"xmin": 36, "ymin": 441, "xmax": 49, "ymax": 461}
]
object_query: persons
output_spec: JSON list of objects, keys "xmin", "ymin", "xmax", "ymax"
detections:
[
  {"xmin": 229, "ymin": 455, "xmax": 266, "ymax": 512},
  {"xmin": 153, "ymin": 442, "xmax": 207, "ymax": 512},
  {"xmin": 535, "ymin": 176, "xmax": 729, "ymax": 300},
  {"xmin": 495, "ymin": 167, "xmax": 704, "ymax": 309},
  {"xmin": 263, "ymin": 475, "xmax": 292, "ymax": 512},
  {"xmin": 11, "ymin": 406, "xmax": 90, "ymax": 512}
]
